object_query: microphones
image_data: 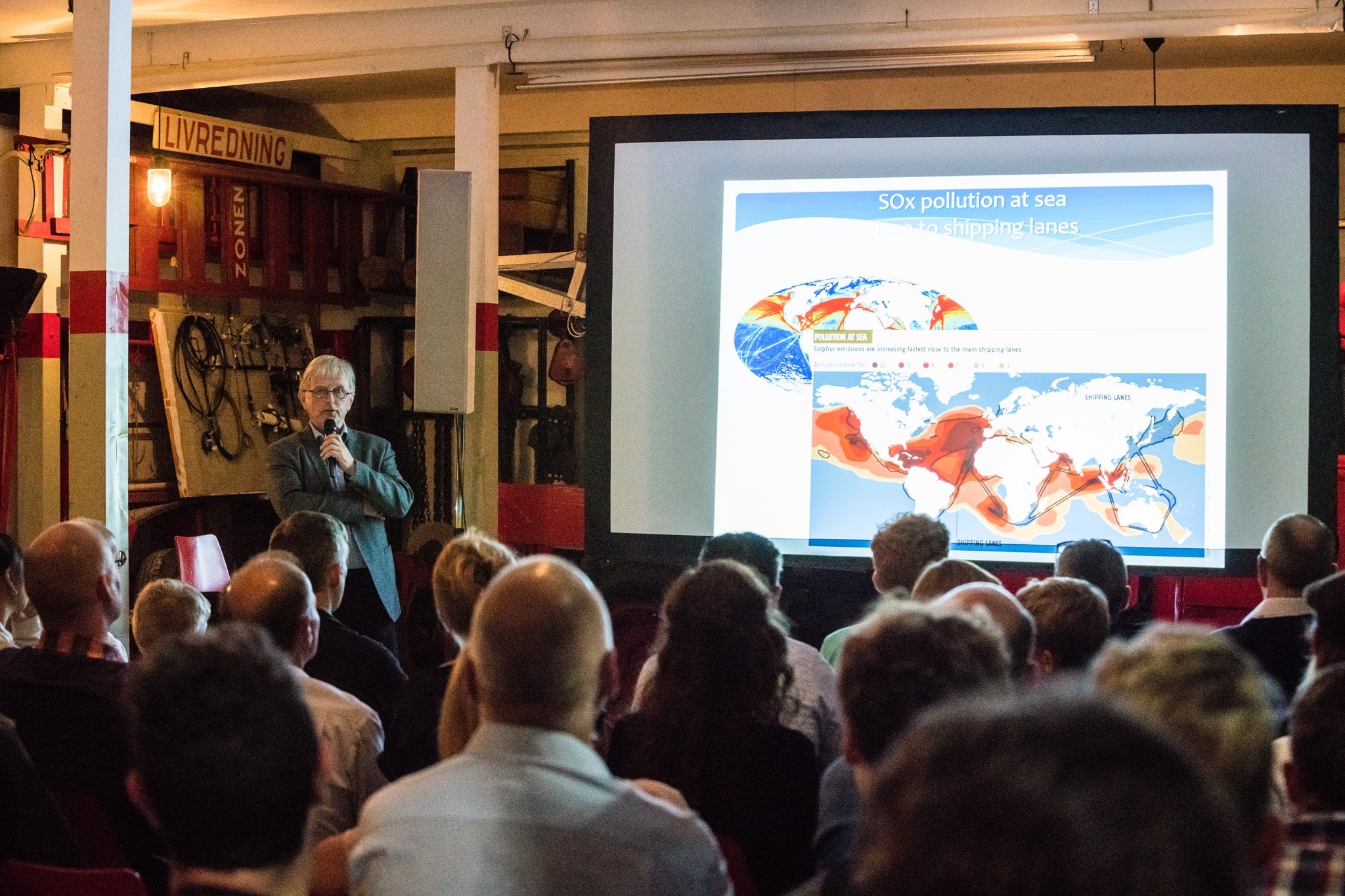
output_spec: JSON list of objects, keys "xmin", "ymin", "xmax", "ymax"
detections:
[{"xmin": 324, "ymin": 419, "xmax": 338, "ymax": 478}]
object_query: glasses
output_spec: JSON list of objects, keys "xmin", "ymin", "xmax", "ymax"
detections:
[
  {"xmin": 115, "ymin": 550, "xmax": 126, "ymax": 566},
  {"xmin": 306, "ymin": 389, "xmax": 349, "ymax": 399},
  {"xmin": 1055, "ymin": 539, "xmax": 1113, "ymax": 562}
]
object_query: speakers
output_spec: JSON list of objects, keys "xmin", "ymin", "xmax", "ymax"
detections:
[{"xmin": 414, "ymin": 170, "xmax": 482, "ymax": 414}]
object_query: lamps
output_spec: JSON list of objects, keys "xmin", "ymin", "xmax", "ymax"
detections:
[{"xmin": 147, "ymin": 106, "xmax": 172, "ymax": 207}]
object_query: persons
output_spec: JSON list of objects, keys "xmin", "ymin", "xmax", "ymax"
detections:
[
  {"xmin": 808, "ymin": 510, "xmax": 1038, "ymax": 872},
  {"xmin": 0, "ymin": 517, "xmax": 171, "ymax": 896},
  {"xmin": 1267, "ymin": 569, "xmax": 1345, "ymax": 895},
  {"xmin": 607, "ymin": 533, "xmax": 842, "ymax": 894},
  {"xmin": 1089, "ymin": 621, "xmax": 1281, "ymax": 823},
  {"xmin": 123, "ymin": 511, "xmax": 436, "ymax": 896},
  {"xmin": 1207, "ymin": 513, "xmax": 1338, "ymax": 741},
  {"xmin": 349, "ymin": 526, "xmax": 735, "ymax": 896},
  {"xmin": 851, "ymin": 670, "xmax": 1255, "ymax": 896},
  {"xmin": 1013, "ymin": 537, "xmax": 1145, "ymax": 684},
  {"xmin": 266, "ymin": 355, "xmax": 414, "ymax": 657}
]
